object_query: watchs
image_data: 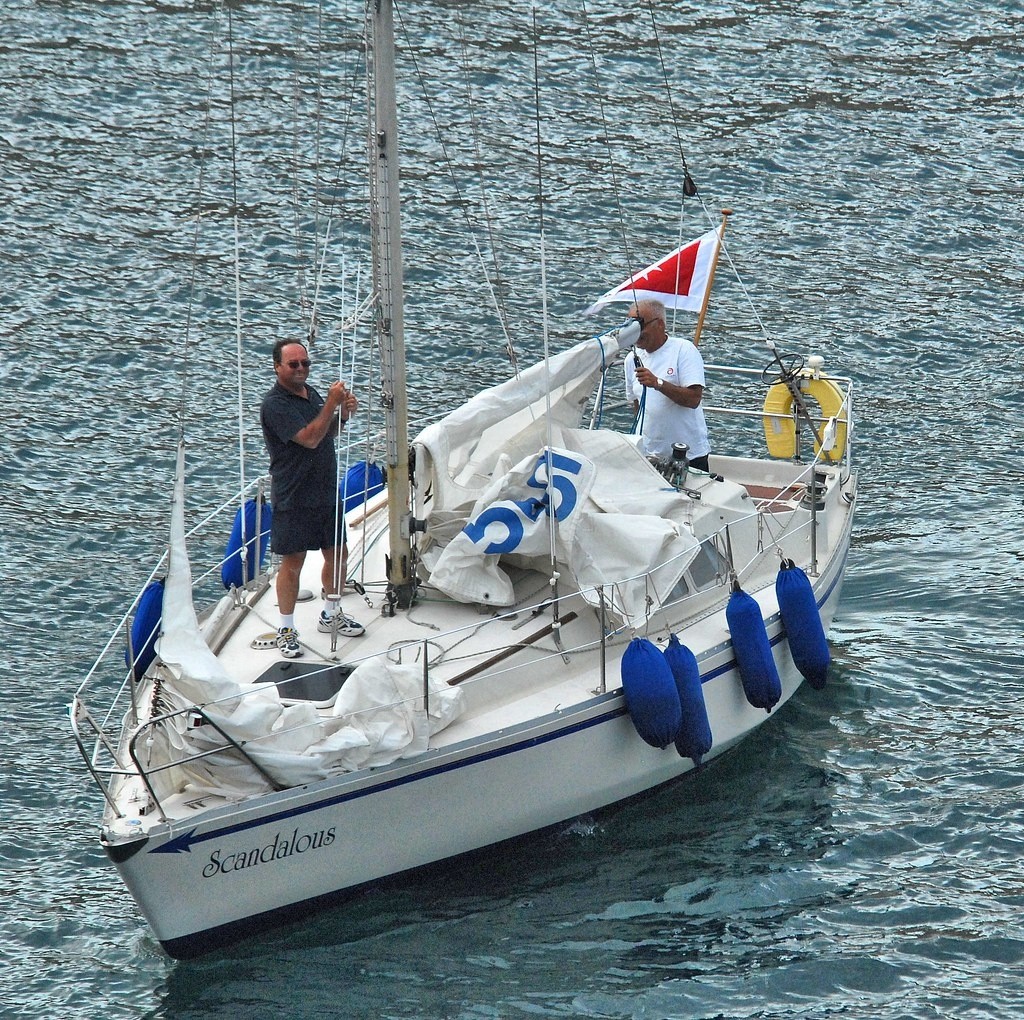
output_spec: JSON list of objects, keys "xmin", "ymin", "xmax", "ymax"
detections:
[{"xmin": 655, "ymin": 378, "xmax": 663, "ymax": 391}]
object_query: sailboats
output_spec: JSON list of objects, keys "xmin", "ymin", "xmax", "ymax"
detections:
[{"xmin": 68, "ymin": 1, "xmax": 867, "ymax": 960}]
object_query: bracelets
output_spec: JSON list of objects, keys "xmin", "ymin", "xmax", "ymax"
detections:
[{"xmin": 335, "ymin": 412, "xmax": 348, "ymax": 424}]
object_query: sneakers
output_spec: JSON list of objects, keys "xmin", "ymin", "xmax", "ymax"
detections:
[
  {"xmin": 317, "ymin": 608, "xmax": 366, "ymax": 636},
  {"xmin": 276, "ymin": 627, "xmax": 304, "ymax": 657}
]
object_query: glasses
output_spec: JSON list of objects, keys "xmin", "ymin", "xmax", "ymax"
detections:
[{"xmin": 281, "ymin": 358, "xmax": 312, "ymax": 368}]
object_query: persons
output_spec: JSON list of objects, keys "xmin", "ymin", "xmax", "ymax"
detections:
[
  {"xmin": 623, "ymin": 300, "xmax": 712, "ymax": 473},
  {"xmin": 259, "ymin": 338, "xmax": 365, "ymax": 658}
]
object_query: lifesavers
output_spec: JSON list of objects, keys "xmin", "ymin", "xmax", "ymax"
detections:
[{"xmin": 763, "ymin": 367, "xmax": 848, "ymax": 461}]
